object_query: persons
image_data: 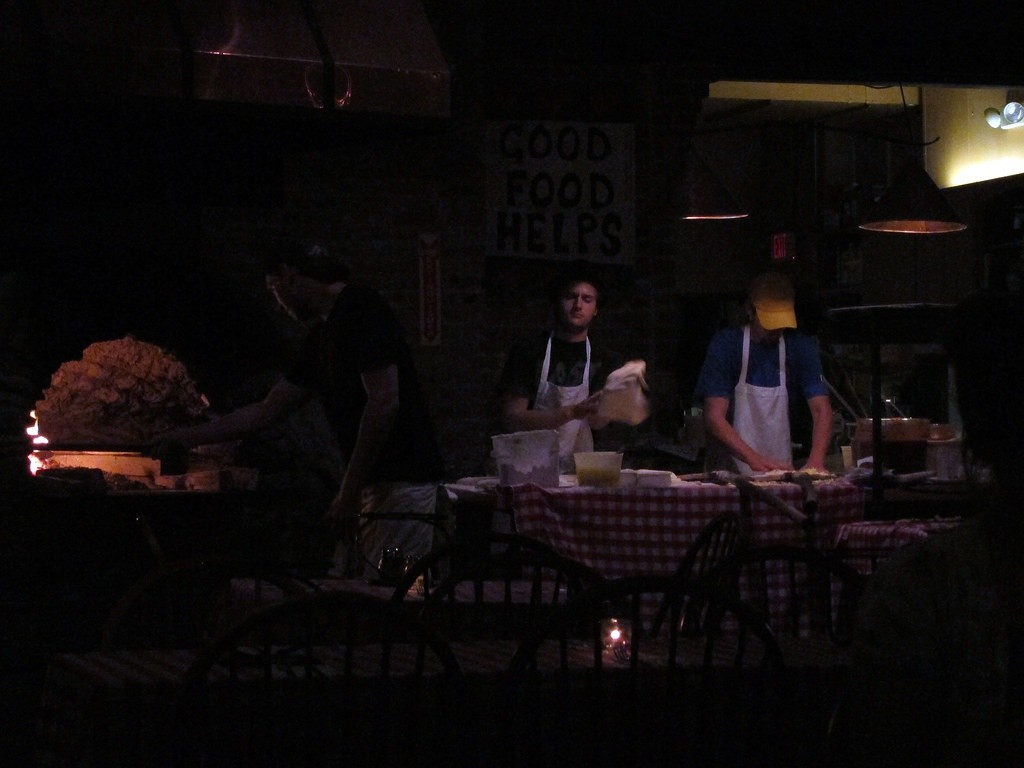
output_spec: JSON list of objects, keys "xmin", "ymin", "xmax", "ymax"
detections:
[
  {"xmin": 497, "ymin": 281, "xmax": 610, "ymax": 474},
  {"xmin": 138, "ymin": 237, "xmax": 444, "ymax": 582},
  {"xmin": 694, "ymin": 271, "xmax": 831, "ymax": 473}
]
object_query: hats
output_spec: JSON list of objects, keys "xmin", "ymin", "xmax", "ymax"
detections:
[{"xmin": 748, "ymin": 274, "xmax": 801, "ymax": 332}]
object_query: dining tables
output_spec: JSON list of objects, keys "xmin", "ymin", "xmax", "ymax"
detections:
[{"xmin": 0, "ymin": 440, "xmax": 1022, "ymax": 766}]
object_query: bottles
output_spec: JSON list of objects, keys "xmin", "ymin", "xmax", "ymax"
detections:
[{"xmin": 377, "ymin": 544, "xmax": 422, "ymax": 595}]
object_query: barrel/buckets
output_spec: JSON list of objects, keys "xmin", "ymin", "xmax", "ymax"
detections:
[
  {"xmin": 490, "ymin": 428, "xmax": 560, "ymax": 487},
  {"xmin": 571, "ymin": 450, "xmax": 625, "ymax": 489}
]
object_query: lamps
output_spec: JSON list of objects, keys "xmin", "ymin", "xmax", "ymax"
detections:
[
  {"xmin": 986, "ymin": 100, "xmax": 1024, "ymax": 133},
  {"xmin": 853, "ymin": 159, "xmax": 969, "ymax": 234}
]
